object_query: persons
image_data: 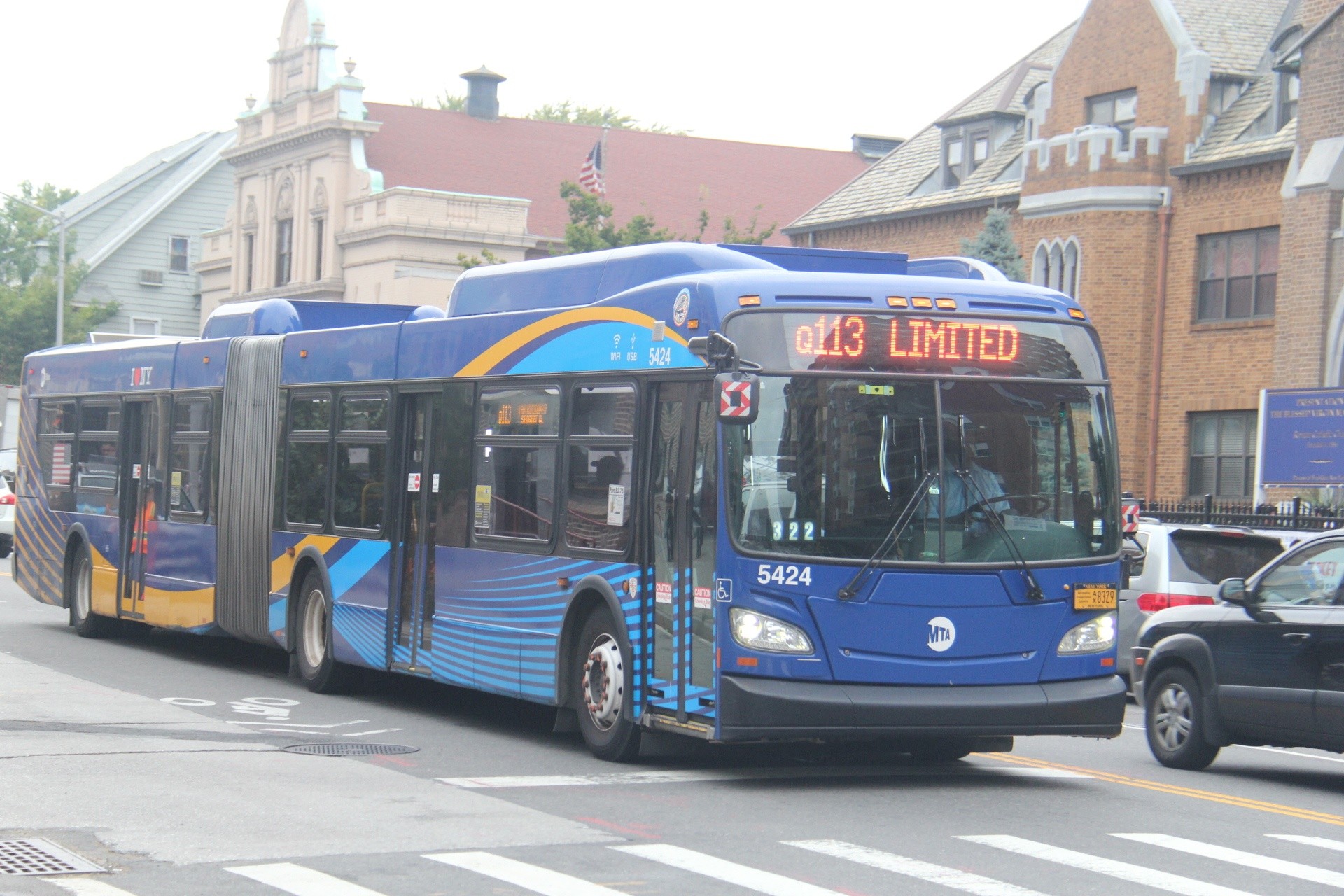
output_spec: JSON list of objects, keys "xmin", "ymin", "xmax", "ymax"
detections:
[
  {"xmin": 577, "ymin": 455, "xmax": 625, "ymax": 499},
  {"xmin": 920, "ymin": 419, "xmax": 1017, "ymax": 539},
  {"xmin": 97, "ymin": 443, "xmax": 118, "ymax": 479},
  {"xmin": 1310, "ymin": 588, "xmax": 1343, "ymax": 605}
]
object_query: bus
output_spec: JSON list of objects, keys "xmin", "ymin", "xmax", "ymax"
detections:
[{"xmin": 10, "ymin": 244, "xmax": 1146, "ymax": 763}]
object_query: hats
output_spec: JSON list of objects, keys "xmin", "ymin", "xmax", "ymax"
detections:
[{"xmin": 590, "ymin": 455, "xmax": 624, "ymax": 471}]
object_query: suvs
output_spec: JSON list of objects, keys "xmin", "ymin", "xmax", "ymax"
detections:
[{"xmin": 1132, "ymin": 527, "xmax": 1344, "ymax": 771}]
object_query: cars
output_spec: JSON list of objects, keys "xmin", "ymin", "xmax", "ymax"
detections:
[
  {"xmin": 1059, "ymin": 517, "xmax": 1288, "ymax": 677},
  {"xmin": 690, "ymin": 454, "xmax": 827, "ymax": 513},
  {"xmin": 0, "ymin": 470, "xmax": 17, "ymax": 558}
]
